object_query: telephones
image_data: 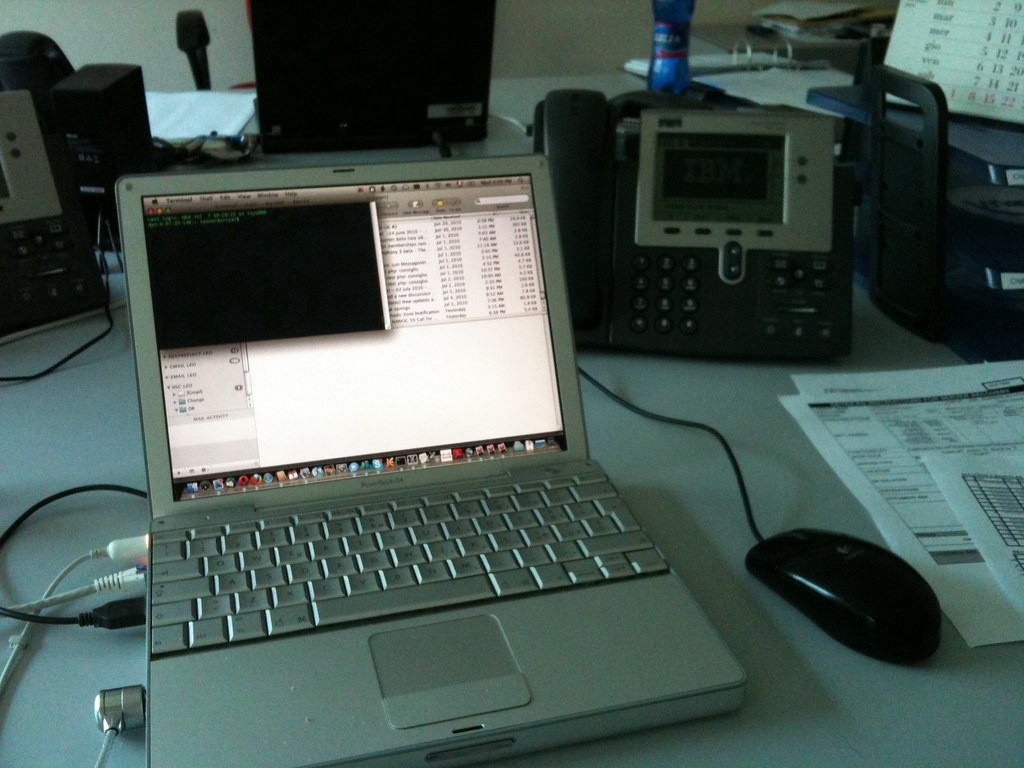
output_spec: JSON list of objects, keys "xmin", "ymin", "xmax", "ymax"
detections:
[
  {"xmin": 0, "ymin": 85, "xmax": 108, "ymax": 343},
  {"xmin": 534, "ymin": 85, "xmax": 858, "ymax": 371}
]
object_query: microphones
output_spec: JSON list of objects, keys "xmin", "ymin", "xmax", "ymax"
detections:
[{"xmin": 174, "ymin": 9, "xmax": 214, "ymax": 91}]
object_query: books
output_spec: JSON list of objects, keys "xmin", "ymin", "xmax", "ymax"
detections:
[{"xmin": 617, "ymin": 3, "xmax": 854, "ymax": 106}]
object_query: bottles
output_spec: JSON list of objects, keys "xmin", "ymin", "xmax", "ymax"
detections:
[{"xmin": 648, "ymin": 0, "xmax": 696, "ymax": 95}]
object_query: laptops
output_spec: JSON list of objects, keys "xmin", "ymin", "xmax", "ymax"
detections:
[
  {"xmin": 250, "ymin": 0, "xmax": 496, "ymax": 153},
  {"xmin": 111, "ymin": 152, "xmax": 750, "ymax": 768}
]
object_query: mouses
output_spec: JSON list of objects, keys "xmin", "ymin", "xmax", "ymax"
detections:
[{"xmin": 745, "ymin": 527, "xmax": 943, "ymax": 666}]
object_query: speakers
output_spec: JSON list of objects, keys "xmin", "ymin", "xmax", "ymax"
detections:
[{"xmin": 0, "ymin": 31, "xmax": 159, "ymax": 251}]
